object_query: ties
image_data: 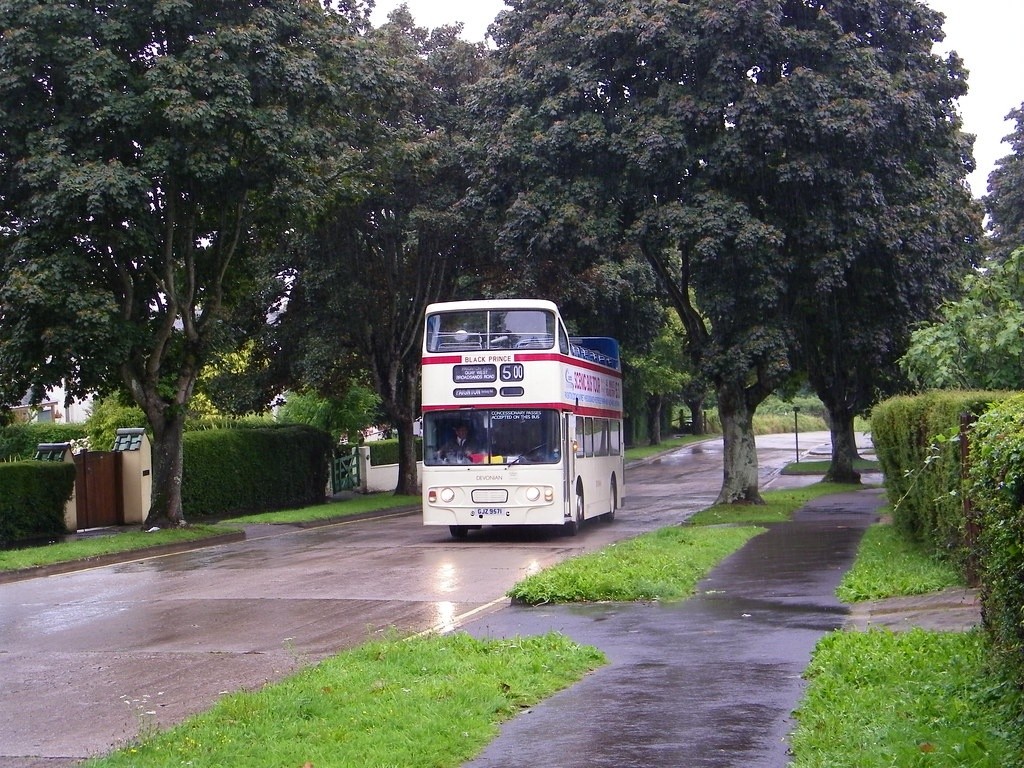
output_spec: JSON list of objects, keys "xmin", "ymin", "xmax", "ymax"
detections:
[{"xmin": 460, "ymin": 439, "xmax": 464, "ymax": 446}]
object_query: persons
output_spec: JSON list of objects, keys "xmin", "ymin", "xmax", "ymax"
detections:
[{"xmin": 435, "ymin": 422, "xmax": 471, "ymax": 457}]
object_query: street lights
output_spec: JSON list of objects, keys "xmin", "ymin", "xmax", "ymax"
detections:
[{"xmin": 792, "ymin": 407, "xmax": 801, "ymax": 462}]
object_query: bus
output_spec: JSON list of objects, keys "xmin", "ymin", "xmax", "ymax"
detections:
[{"xmin": 422, "ymin": 299, "xmax": 625, "ymax": 539}]
